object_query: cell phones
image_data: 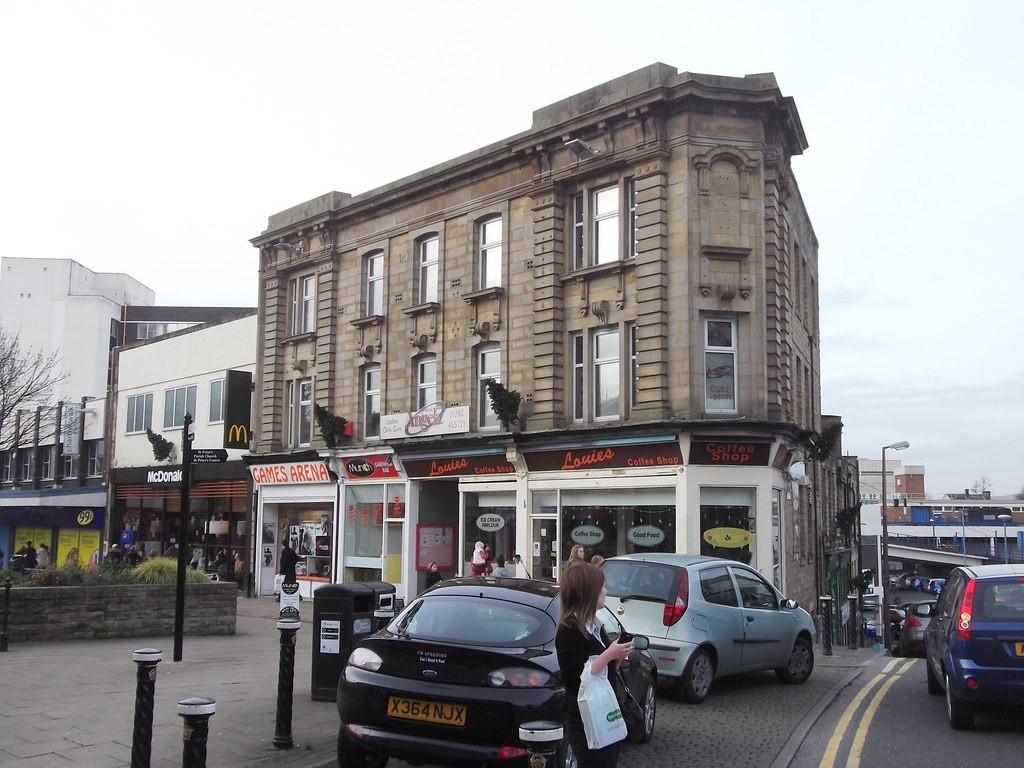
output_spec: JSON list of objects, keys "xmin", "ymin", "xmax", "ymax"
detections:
[{"xmin": 617, "ymin": 632, "xmax": 634, "ymax": 644}]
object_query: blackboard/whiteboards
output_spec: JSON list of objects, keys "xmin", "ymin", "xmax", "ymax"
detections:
[{"xmin": 394, "ymin": 599, "xmax": 404, "ymax": 617}]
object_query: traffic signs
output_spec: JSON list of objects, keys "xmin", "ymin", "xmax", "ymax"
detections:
[{"xmin": 190, "ymin": 449, "xmax": 228, "ymax": 464}]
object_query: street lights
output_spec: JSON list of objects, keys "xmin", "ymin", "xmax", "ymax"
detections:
[{"xmin": 881, "ymin": 441, "xmax": 910, "ymax": 650}]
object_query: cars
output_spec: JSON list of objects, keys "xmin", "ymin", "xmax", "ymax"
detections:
[
  {"xmin": 335, "ymin": 576, "xmax": 658, "ymax": 768},
  {"xmin": 863, "ymin": 619, "xmax": 894, "ymax": 637},
  {"xmin": 889, "ymin": 571, "xmax": 946, "ymax": 594}
]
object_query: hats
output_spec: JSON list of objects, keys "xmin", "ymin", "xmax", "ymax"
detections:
[{"xmin": 475, "ymin": 541, "xmax": 484, "ymax": 548}]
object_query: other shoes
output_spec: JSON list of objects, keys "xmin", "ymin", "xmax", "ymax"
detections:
[
  {"xmin": 275, "ymin": 599, "xmax": 279, "ymax": 602},
  {"xmin": 299, "ymin": 596, "xmax": 302, "ymax": 601}
]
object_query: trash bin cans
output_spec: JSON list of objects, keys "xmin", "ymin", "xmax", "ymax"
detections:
[{"xmin": 312, "ymin": 580, "xmax": 396, "ymax": 704}]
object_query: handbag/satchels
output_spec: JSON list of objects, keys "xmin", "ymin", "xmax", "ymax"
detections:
[
  {"xmin": 577, "ymin": 655, "xmax": 628, "ymax": 750},
  {"xmin": 273, "ymin": 574, "xmax": 286, "ymax": 593}
]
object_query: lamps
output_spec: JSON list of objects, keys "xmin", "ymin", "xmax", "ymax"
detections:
[
  {"xmin": 273, "ymin": 243, "xmax": 300, "ymax": 256},
  {"xmin": 205, "ymin": 520, "xmax": 229, "ymax": 537},
  {"xmin": 77, "ymin": 408, "xmax": 96, "ymax": 414},
  {"xmin": 564, "ymin": 138, "xmax": 597, "ymax": 159},
  {"xmin": 237, "ymin": 521, "xmax": 246, "ymax": 536}
]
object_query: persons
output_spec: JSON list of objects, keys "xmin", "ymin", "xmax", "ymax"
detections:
[
  {"xmin": 471, "ymin": 541, "xmax": 509, "ymax": 578},
  {"xmin": 16, "ymin": 540, "xmax": 243, "ymax": 573},
  {"xmin": 568, "ymin": 544, "xmax": 604, "ymax": 567},
  {"xmin": 555, "ymin": 561, "xmax": 635, "ymax": 768},
  {"xmin": 276, "ymin": 539, "xmax": 303, "ymax": 602},
  {"xmin": 264, "ymin": 548, "xmax": 272, "ymax": 566},
  {"xmin": 426, "ymin": 561, "xmax": 443, "ymax": 589},
  {"xmin": 893, "ymin": 593, "xmax": 902, "ymax": 605}
]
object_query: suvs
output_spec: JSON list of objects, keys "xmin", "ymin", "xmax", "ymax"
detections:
[
  {"xmin": 912, "ymin": 564, "xmax": 1024, "ymax": 729},
  {"xmin": 595, "ymin": 553, "xmax": 817, "ymax": 704},
  {"xmin": 899, "ymin": 600, "xmax": 939, "ymax": 657}
]
